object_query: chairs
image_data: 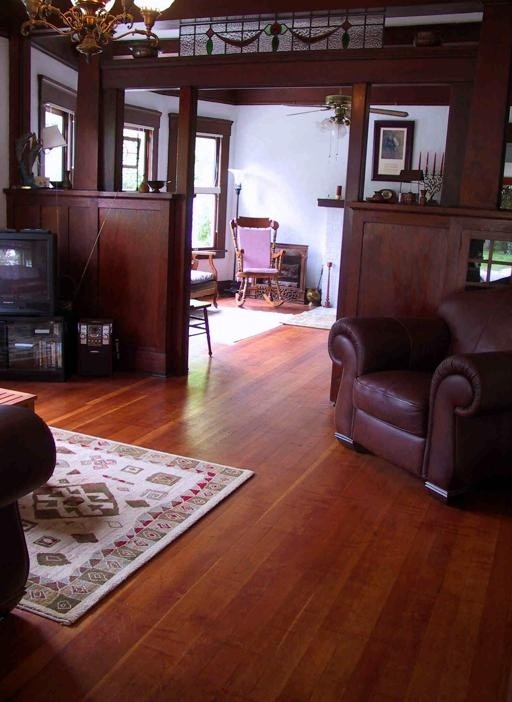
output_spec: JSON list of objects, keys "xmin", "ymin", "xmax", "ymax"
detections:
[
  {"xmin": 327, "ymin": 283, "xmax": 511, "ymax": 502},
  {"xmin": 190, "ymin": 250, "xmax": 220, "ymax": 308},
  {"xmin": 230, "ymin": 215, "xmax": 285, "ymax": 309}
]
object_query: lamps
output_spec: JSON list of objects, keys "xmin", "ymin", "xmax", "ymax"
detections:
[
  {"xmin": 21, "ymin": 1, "xmax": 174, "ymax": 66},
  {"xmin": 319, "ymin": 114, "xmax": 350, "ymax": 140},
  {"xmin": 17, "ymin": 124, "xmax": 67, "ymax": 190}
]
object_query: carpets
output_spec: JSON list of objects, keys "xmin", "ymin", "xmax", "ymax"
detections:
[{"xmin": 17, "ymin": 424, "xmax": 255, "ymax": 628}]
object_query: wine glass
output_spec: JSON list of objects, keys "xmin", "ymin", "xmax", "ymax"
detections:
[{"xmin": 148, "ymin": 181, "xmax": 165, "ymax": 192}]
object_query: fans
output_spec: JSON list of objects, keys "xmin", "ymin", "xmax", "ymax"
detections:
[{"xmin": 283, "ymin": 87, "xmax": 408, "ymax": 161}]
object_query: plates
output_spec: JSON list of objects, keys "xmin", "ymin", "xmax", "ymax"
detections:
[{"xmin": 380, "ymin": 189, "xmax": 394, "ymax": 202}]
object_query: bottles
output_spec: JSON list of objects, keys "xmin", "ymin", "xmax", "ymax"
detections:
[
  {"xmin": 139, "ymin": 174, "xmax": 149, "ymax": 192},
  {"xmin": 61, "ymin": 171, "xmax": 72, "ymax": 190},
  {"xmin": 335, "ymin": 186, "xmax": 342, "ymax": 199},
  {"xmin": 419, "ymin": 190, "xmax": 427, "ymax": 205},
  {"xmin": 499, "ymin": 178, "xmax": 511, "ymax": 210}
]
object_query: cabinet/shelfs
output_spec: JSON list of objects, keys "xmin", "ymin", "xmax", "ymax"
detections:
[{"xmin": 0, "ymin": 313, "xmax": 70, "ymax": 384}]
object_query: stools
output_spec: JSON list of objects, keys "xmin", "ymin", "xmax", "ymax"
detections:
[{"xmin": 190, "ymin": 299, "xmax": 213, "ymax": 357}]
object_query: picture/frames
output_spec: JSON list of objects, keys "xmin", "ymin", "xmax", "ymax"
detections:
[{"xmin": 370, "ymin": 119, "xmax": 415, "ymax": 184}]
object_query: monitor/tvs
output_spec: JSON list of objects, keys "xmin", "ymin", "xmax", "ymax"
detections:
[{"xmin": 0, "ymin": 231, "xmax": 57, "ymax": 316}]
